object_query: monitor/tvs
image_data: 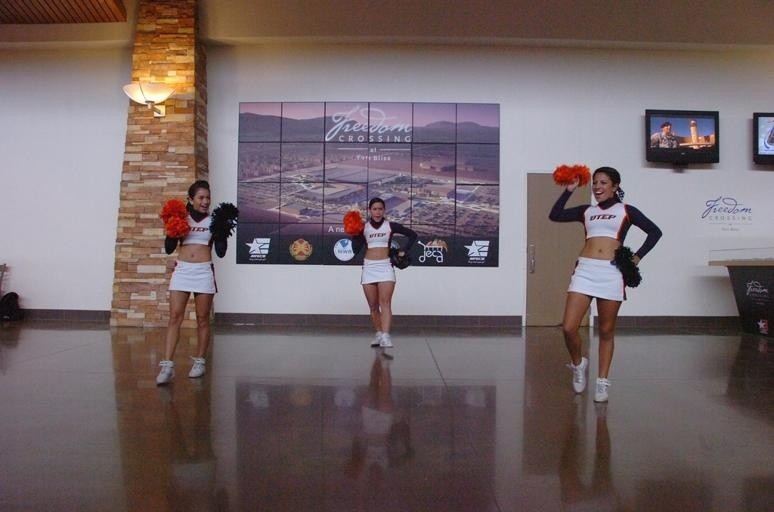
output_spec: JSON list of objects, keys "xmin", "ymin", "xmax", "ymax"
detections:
[
  {"xmin": 752, "ymin": 112, "xmax": 774, "ymax": 166},
  {"xmin": 645, "ymin": 109, "xmax": 719, "ymax": 169}
]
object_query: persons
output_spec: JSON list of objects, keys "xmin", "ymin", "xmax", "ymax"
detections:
[
  {"xmin": 162, "ymin": 375, "xmax": 229, "ymax": 512},
  {"xmin": 351, "ymin": 197, "xmax": 417, "ymax": 348},
  {"xmin": 155, "ymin": 179, "xmax": 228, "ymax": 385},
  {"xmin": 548, "ymin": 167, "xmax": 662, "ymax": 403},
  {"xmin": 343, "ymin": 345, "xmax": 416, "ymax": 512},
  {"xmin": 650, "ymin": 121, "xmax": 680, "ymax": 150},
  {"xmin": 557, "ymin": 393, "xmax": 620, "ymax": 512}
]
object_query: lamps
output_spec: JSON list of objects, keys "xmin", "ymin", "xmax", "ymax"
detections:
[{"xmin": 121, "ymin": 81, "xmax": 177, "ymax": 118}]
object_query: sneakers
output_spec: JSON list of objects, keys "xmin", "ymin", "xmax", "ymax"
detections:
[
  {"xmin": 189, "ymin": 358, "xmax": 207, "ymax": 378},
  {"xmin": 371, "ymin": 335, "xmax": 394, "ymax": 348},
  {"xmin": 594, "ymin": 378, "xmax": 610, "ymax": 403},
  {"xmin": 155, "ymin": 360, "xmax": 175, "ymax": 385},
  {"xmin": 571, "ymin": 357, "xmax": 588, "ymax": 393}
]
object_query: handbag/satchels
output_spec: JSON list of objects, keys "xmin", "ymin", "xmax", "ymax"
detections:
[{"xmin": 0, "ymin": 292, "xmax": 23, "ymax": 322}]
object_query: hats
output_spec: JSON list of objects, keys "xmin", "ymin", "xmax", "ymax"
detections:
[{"xmin": 661, "ymin": 122, "xmax": 669, "ymax": 128}]
